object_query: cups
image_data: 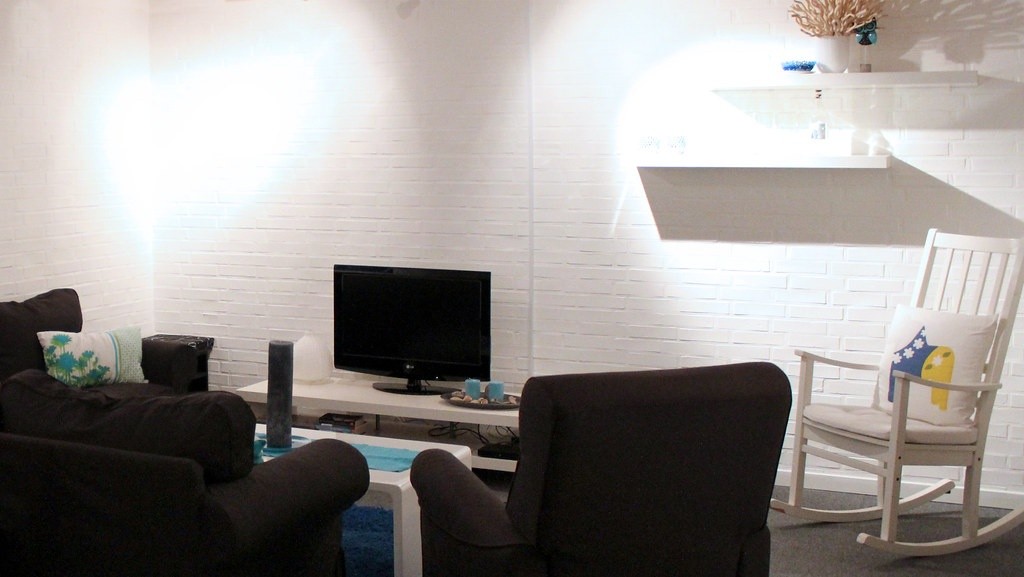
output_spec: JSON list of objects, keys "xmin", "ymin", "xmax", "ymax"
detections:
[
  {"xmin": 465, "ymin": 379, "xmax": 480, "ymax": 400},
  {"xmin": 488, "ymin": 382, "xmax": 504, "ymax": 402}
]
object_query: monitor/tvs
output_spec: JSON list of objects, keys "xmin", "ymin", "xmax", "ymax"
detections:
[{"xmin": 333, "ymin": 264, "xmax": 491, "ymax": 395}]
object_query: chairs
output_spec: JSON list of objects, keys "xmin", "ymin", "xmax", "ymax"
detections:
[{"xmin": 770, "ymin": 227, "xmax": 1024, "ymax": 557}]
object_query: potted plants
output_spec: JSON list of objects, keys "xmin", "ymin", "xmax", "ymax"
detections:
[{"xmin": 786, "ymin": 0, "xmax": 887, "ymax": 72}]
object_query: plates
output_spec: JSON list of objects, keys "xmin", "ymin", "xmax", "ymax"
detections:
[{"xmin": 440, "ymin": 392, "xmax": 522, "ymax": 408}]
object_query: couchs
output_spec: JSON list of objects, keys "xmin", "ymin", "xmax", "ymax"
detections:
[
  {"xmin": 409, "ymin": 362, "xmax": 792, "ymax": 576},
  {"xmin": 0, "ymin": 288, "xmax": 201, "ymax": 394},
  {"xmin": 0, "ymin": 368, "xmax": 369, "ymax": 576}
]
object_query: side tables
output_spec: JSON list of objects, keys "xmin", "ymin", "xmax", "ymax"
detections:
[{"xmin": 141, "ymin": 333, "xmax": 214, "ymax": 393}]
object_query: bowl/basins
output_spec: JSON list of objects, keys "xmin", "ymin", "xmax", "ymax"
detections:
[{"xmin": 780, "ymin": 58, "xmax": 816, "ymax": 71}]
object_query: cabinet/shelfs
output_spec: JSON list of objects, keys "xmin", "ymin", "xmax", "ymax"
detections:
[
  {"xmin": 635, "ymin": 70, "xmax": 981, "ymax": 168},
  {"xmin": 237, "ymin": 374, "xmax": 522, "ymax": 472}
]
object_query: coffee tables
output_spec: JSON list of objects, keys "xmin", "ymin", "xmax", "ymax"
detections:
[{"xmin": 253, "ymin": 422, "xmax": 473, "ymax": 576}]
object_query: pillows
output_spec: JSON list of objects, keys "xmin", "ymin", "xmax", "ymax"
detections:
[
  {"xmin": 35, "ymin": 326, "xmax": 150, "ymax": 386},
  {"xmin": 870, "ymin": 302, "xmax": 999, "ymax": 428}
]
